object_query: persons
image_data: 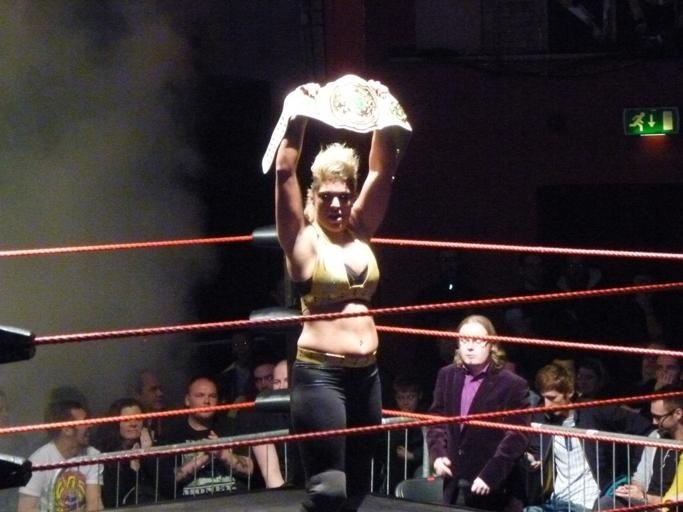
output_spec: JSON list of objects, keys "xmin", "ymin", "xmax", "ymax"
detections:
[
  {"xmin": 392, "ymin": 313, "xmax": 683, "ymax": 512},
  {"xmin": 21, "ymin": 354, "xmax": 294, "ymax": 510},
  {"xmin": 271, "ymin": 80, "xmax": 400, "ymax": 510}
]
